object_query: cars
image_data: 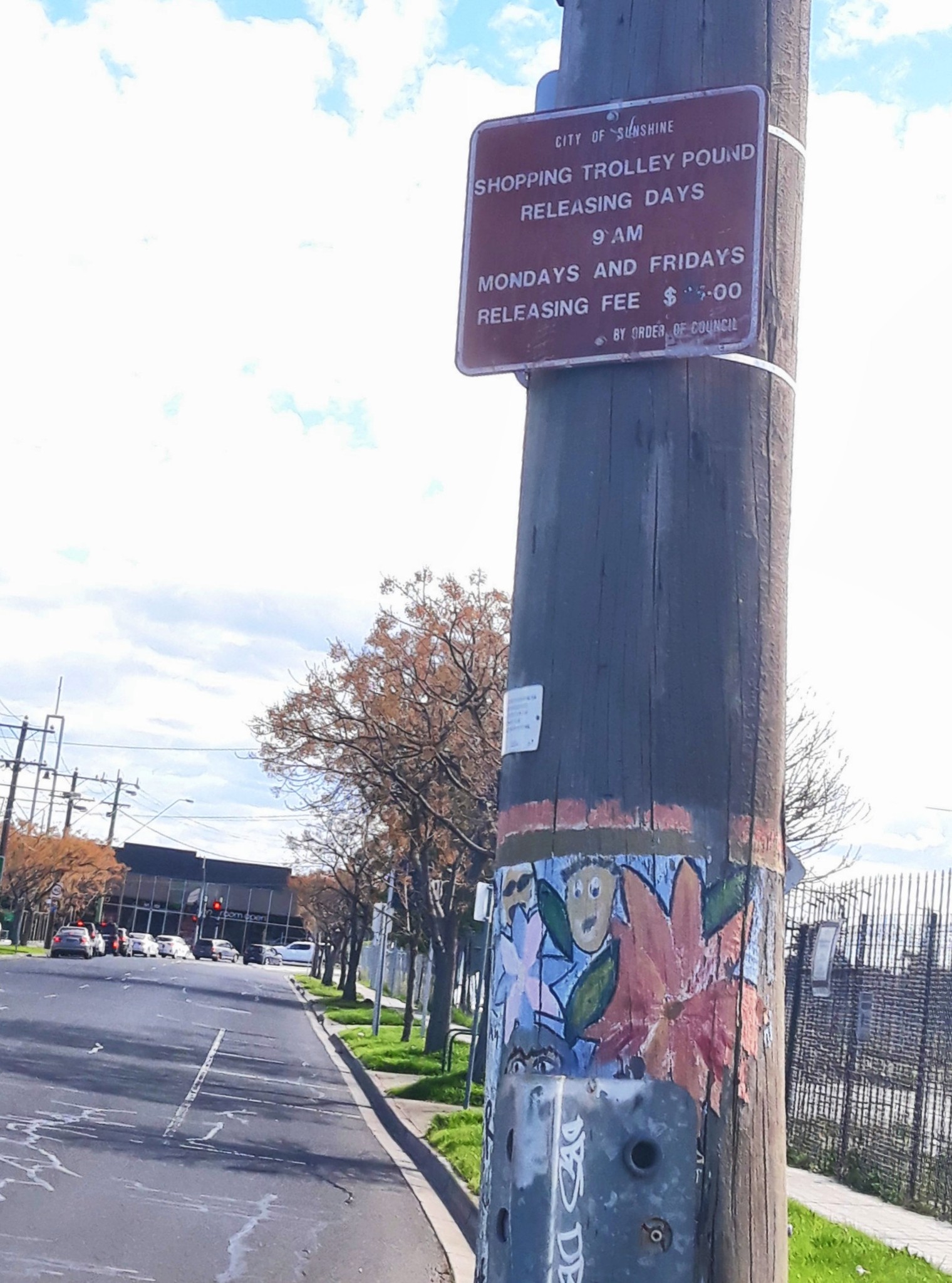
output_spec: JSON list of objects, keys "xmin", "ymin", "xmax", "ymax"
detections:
[
  {"xmin": 242, "ymin": 944, "xmax": 282, "ymax": 966},
  {"xmin": 66, "ymin": 921, "xmax": 106, "ymax": 957},
  {"xmin": 49, "ymin": 927, "xmax": 93, "ymax": 959},
  {"xmin": 155, "ymin": 935, "xmax": 191, "ymax": 959},
  {"xmin": 128, "ymin": 933, "xmax": 159, "ymax": 958},
  {"xmin": 118, "ymin": 928, "xmax": 134, "ymax": 958},
  {"xmin": 192, "ymin": 938, "xmax": 240, "ymax": 963}
]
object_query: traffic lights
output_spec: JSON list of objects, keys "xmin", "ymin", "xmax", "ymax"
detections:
[
  {"xmin": 213, "ymin": 901, "xmax": 222, "ymax": 910},
  {"xmin": 192, "ymin": 916, "xmax": 197, "ymax": 923}
]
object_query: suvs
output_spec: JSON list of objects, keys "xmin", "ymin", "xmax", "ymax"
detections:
[
  {"xmin": 94, "ymin": 922, "xmax": 120, "ymax": 956},
  {"xmin": 272, "ymin": 941, "xmax": 326, "ymax": 963}
]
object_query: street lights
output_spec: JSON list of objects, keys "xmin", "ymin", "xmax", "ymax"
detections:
[
  {"xmin": 96, "ymin": 799, "xmax": 194, "ymax": 922},
  {"xmin": 0, "ymin": 805, "xmax": 88, "ymax": 885},
  {"xmin": 45, "ymin": 788, "xmax": 136, "ymax": 949}
]
